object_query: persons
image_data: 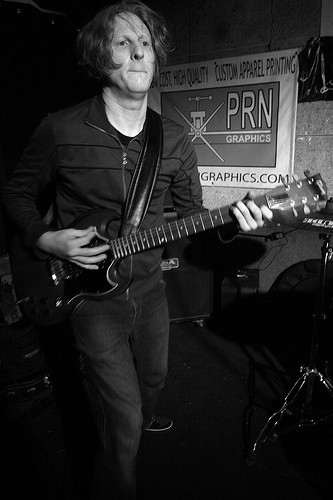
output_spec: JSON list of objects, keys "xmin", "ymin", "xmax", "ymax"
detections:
[{"xmin": 0, "ymin": 0, "xmax": 273, "ymax": 500}]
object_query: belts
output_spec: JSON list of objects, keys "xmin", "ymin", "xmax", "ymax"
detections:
[{"xmin": 0, "ymin": 374, "xmax": 53, "ymax": 400}]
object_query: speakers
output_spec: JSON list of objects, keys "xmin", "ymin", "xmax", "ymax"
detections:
[{"xmin": 158, "ymin": 256, "xmax": 212, "ymax": 323}]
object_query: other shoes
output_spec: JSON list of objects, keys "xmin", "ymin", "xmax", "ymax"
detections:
[{"xmin": 140, "ymin": 414, "xmax": 174, "ymax": 431}]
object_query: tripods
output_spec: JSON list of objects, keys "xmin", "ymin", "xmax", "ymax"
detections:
[{"xmin": 251, "ymin": 233, "xmax": 333, "ymax": 458}]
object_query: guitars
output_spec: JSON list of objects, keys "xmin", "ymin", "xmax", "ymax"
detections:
[{"xmin": 27, "ymin": 169, "xmax": 329, "ymax": 317}]
